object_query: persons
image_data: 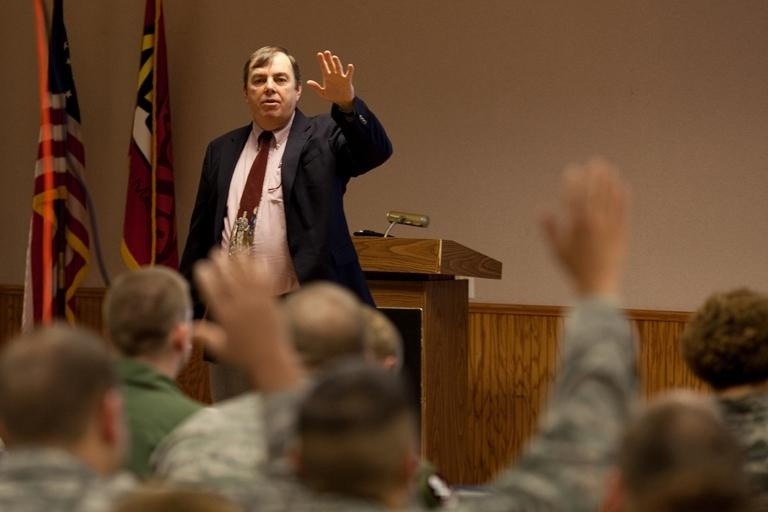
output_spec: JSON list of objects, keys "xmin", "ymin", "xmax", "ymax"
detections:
[
  {"xmin": 0, "ymin": 160, "xmax": 768, "ymax": 512},
  {"xmin": 178, "ymin": 45, "xmax": 392, "ymax": 406}
]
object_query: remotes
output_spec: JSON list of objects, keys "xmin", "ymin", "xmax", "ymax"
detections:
[{"xmin": 353, "ymin": 229, "xmax": 395, "ymax": 237}]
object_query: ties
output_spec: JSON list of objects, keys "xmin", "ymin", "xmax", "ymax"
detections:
[{"xmin": 228, "ymin": 128, "xmax": 273, "ymax": 248}]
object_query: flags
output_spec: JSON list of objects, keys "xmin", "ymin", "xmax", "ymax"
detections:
[
  {"xmin": 20, "ymin": 0, "xmax": 95, "ymax": 331},
  {"xmin": 121, "ymin": 0, "xmax": 179, "ymax": 272}
]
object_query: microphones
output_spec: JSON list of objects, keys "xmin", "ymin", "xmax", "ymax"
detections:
[{"xmin": 385, "ymin": 210, "xmax": 430, "ymax": 227}]
